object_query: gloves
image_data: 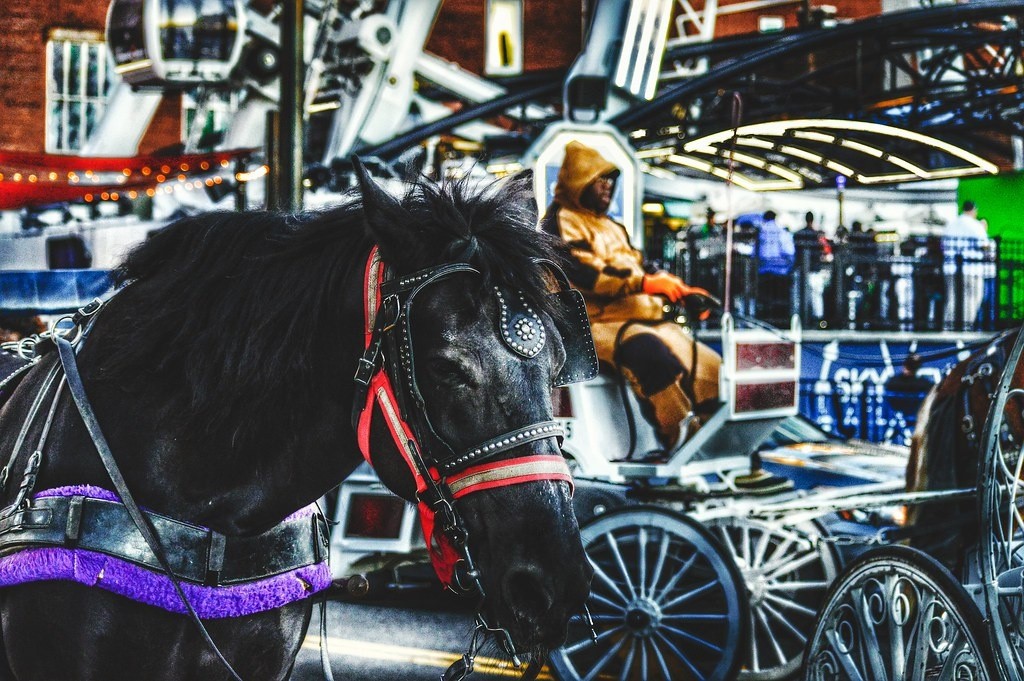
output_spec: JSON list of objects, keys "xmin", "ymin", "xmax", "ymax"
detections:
[
  {"xmin": 643, "ymin": 272, "xmax": 689, "ymax": 302},
  {"xmin": 678, "ymin": 285, "xmax": 711, "ymax": 320}
]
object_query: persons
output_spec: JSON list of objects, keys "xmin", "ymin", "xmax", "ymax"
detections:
[
  {"xmin": 536, "ymin": 139, "xmax": 721, "ymax": 451},
  {"xmin": 884, "ymin": 353, "xmax": 935, "ymax": 427},
  {"xmin": 751, "ymin": 211, "xmax": 796, "ymax": 318},
  {"xmin": 834, "ymin": 220, "xmax": 877, "ymax": 291},
  {"xmin": 700, "ymin": 207, "xmax": 718, "ymax": 236},
  {"xmin": 943, "ymin": 200, "xmax": 996, "ymax": 330},
  {"xmin": 793, "ymin": 211, "xmax": 828, "ymax": 317}
]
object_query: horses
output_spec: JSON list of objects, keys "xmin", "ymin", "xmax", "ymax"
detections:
[
  {"xmin": 903, "ymin": 329, "xmax": 1024, "ymax": 589},
  {"xmin": 0, "ymin": 152, "xmax": 595, "ymax": 681}
]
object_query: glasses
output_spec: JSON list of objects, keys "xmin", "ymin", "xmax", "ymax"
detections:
[{"xmin": 595, "ymin": 176, "xmax": 615, "ymax": 187}]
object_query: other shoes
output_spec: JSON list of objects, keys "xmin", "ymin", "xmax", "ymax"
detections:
[{"xmin": 734, "ymin": 471, "xmax": 795, "ymax": 493}]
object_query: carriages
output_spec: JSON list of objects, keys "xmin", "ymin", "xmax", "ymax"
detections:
[{"xmin": 320, "ymin": 122, "xmax": 1024, "ymax": 678}]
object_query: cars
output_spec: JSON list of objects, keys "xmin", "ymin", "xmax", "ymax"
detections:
[{"xmin": 649, "ymin": 412, "xmax": 912, "ymax": 564}]
